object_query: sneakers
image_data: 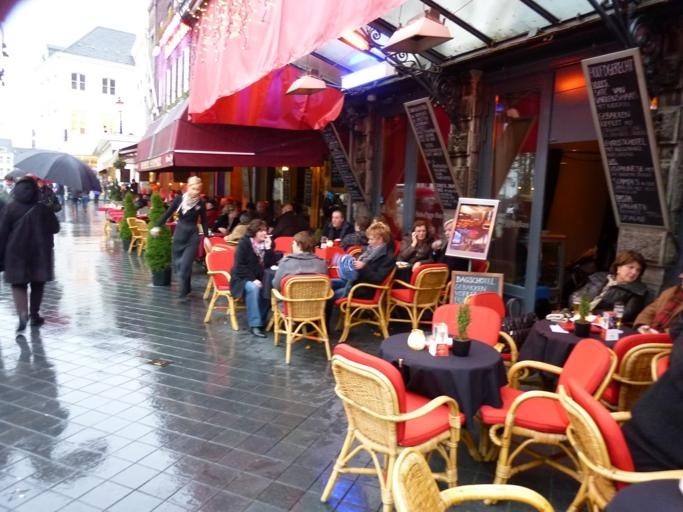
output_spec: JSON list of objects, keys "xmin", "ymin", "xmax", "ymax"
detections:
[
  {"xmin": 29, "ymin": 315, "xmax": 44, "ymax": 325},
  {"xmin": 17, "ymin": 317, "xmax": 28, "ymax": 333}
]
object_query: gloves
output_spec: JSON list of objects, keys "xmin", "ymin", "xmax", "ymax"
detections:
[
  {"xmin": 551, "ymin": 319, "xmax": 568, "ymax": 322},
  {"xmin": 546, "ymin": 308, "xmax": 571, "ymax": 319}
]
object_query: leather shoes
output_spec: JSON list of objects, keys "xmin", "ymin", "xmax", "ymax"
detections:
[{"xmin": 251, "ymin": 327, "xmax": 266, "ymax": 338}]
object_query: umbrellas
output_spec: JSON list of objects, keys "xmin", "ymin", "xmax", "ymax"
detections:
[{"xmin": 14, "ymin": 151, "xmax": 101, "ymax": 192}]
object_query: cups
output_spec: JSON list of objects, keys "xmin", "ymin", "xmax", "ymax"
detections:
[
  {"xmin": 572, "ymin": 291, "xmax": 583, "ymax": 314},
  {"xmin": 434, "ymin": 324, "xmax": 447, "ymax": 347},
  {"xmin": 613, "ymin": 303, "xmax": 624, "ymax": 327}
]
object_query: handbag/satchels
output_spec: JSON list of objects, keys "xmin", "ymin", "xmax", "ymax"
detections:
[
  {"xmin": 501, "ymin": 298, "xmax": 536, "ymax": 353},
  {"xmin": 47, "ymin": 196, "xmax": 62, "ymax": 212}
]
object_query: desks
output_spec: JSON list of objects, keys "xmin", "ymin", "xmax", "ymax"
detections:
[{"xmin": 604, "ymin": 479, "xmax": 682, "ymax": 511}]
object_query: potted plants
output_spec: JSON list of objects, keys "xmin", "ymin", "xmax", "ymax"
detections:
[
  {"xmin": 453, "ymin": 304, "xmax": 472, "ymax": 356},
  {"xmin": 575, "ymin": 296, "xmax": 591, "ymax": 336}
]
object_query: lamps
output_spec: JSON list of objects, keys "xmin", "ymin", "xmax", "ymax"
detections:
[
  {"xmin": 383, "ymin": 4, "xmax": 453, "ymax": 55},
  {"xmin": 286, "ymin": 58, "xmax": 327, "ymax": 95}
]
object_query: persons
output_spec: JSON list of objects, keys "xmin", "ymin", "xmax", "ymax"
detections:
[{"xmin": 0, "ymin": 176, "xmax": 681, "ymax": 511}]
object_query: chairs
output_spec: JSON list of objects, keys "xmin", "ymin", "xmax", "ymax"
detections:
[
  {"xmin": 391, "ymin": 448, "xmax": 555, "ymax": 511},
  {"xmin": 321, "ymin": 342, "xmax": 464, "ymax": 511},
  {"xmin": 607, "ymin": 333, "xmax": 682, "ymax": 408},
  {"xmin": 479, "ymin": 338, "xmax": 618, "ymax": 506},
  {"xmin": 465, "ymin": 290, "xmax": 506, "ymax": 317},
  {"xmin": 427, "ymin": 302, "xmax": 518, "ymax": 389},
  {"xmin": 552, "ymin": 381, "xmax": 682, "ymax": 511}
]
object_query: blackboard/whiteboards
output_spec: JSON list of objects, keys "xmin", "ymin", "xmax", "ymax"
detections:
[
  {"xmin": 450, "ymin": 271, "xmax": 503, "ymax": 305},
  {"xmin": 322, "ymin": 123, "xmax": 368, "ymax": 202},
  {"xmin": 404, "ymin": 95, "xmax": 461, "ymax": 214},
  {"xmin": 581, "ymin": 47, "xmax": 671, "ymax": 229}
]
